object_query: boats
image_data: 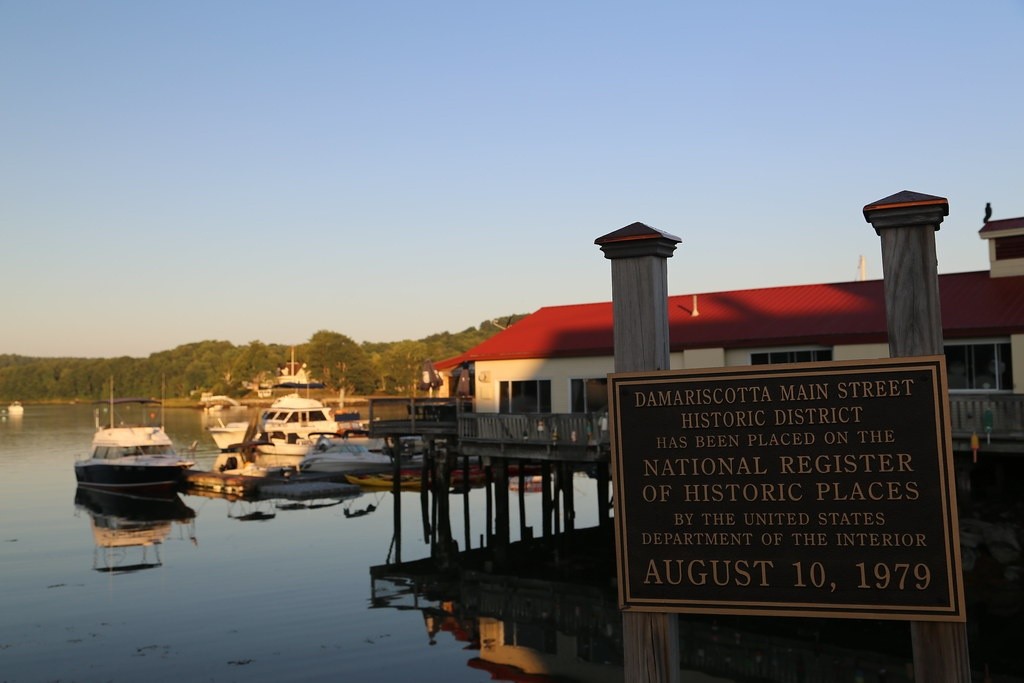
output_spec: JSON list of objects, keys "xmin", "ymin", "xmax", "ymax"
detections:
[
  {"xmin": 203, "ymin": 378, "xmax": 386, "ymax": 457},
  {"xmin": 213, "ymin": 441, "xmax": 295, "ymax": 481},
  {"xmin": 74, "ymin": 483, "xmax": 200, "ymax": 577},
  {"xmin": 74, "ymin": 374, "xmax": 198, "ymax": 490},
  {"xmin": 388, "ymin": 434, "xmax": 478, "ymax": 470},
  {"xmin": 298, "ymin": 431, "xmax": 397, "ymax": 478}
]
